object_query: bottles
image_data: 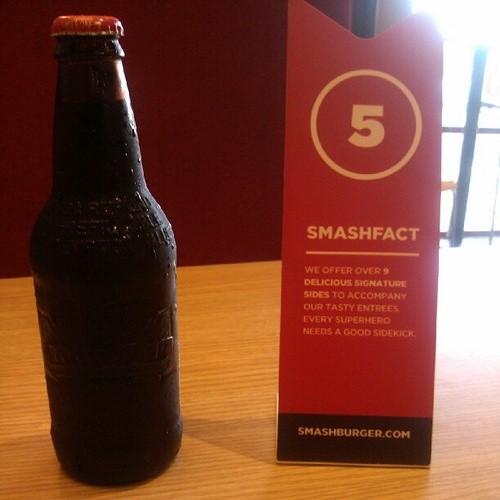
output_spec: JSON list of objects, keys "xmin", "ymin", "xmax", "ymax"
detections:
[{"xmin": 29, "ymin": 13, "xmax": 182, "ymax": 490}]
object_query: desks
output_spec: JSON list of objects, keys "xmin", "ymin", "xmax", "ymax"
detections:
[{"xmin": 0, "ymin": 244, "xmax": 499, "ymax": 499}]
